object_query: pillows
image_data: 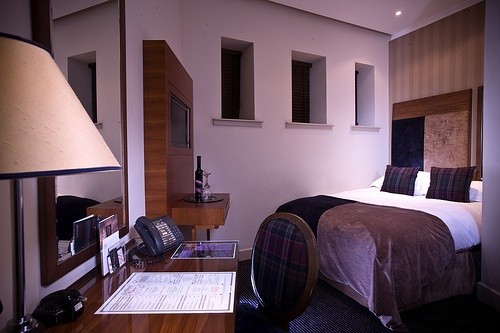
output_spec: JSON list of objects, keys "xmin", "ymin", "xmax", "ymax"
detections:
[
  {"xmin": 427, "ymin": 175, "xmax": 484, "ymax": 202},
  {"xmin": 381, "ymin": 165, "xmax": 421, "ymax": 196},
  {"xmin": 426, "ymin": 163, "xmax": 475, "ymax": 203},
  {"xmin": 370, "ymin": 170, "xmax": 430, "ymax": 196}
]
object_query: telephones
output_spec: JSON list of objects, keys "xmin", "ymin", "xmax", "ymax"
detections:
[{"xmin": 133, "ymin": 214, "xmax": 186, "ymax": 257}]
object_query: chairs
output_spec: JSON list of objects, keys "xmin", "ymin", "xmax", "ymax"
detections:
[{"xmin": 236, "ymin": 212, "xmax": 318, "ymax": 332}]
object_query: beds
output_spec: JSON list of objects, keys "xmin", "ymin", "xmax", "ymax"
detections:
[{"xmin": 271, "ymin": 84, "xmax": 484, "ymax": 332}]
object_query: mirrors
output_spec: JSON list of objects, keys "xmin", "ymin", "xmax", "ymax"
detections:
[{"xmin": 32, "ymin": 0, "xmax": 129, "ymax": 287}]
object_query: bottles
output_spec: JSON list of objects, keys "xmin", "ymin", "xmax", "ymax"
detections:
[{"xmin": 195, "ymin": 155, "xmax": 203, "ymax": 200}]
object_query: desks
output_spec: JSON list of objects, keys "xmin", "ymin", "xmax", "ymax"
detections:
[
  {"xmin": 29, "ymin": 236, "xmax": 241, "ymax": 332},
  {"xmin": 85, "ymin": 197, "xmax": 123, "ymax": 226},
  {"xmin": 173, "ymin": 192, "xmax": 230, "ymax": 242}
]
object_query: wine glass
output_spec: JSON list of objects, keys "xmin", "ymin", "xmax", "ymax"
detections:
[{"xmin": 202, "ymin": 173, "xmax": 211, "ymax": 200}]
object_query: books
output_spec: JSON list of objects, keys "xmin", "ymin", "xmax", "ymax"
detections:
[
  {"xmin": 99, "ymin": 215, "xmax": 118, "ymax": 275},
  {"xmin": 73, "ymin": 214, "xmax": 95, "ymax": 250}
]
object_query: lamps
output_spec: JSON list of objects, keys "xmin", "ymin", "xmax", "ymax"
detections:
[{"xmin": 1, "ymin": 32, "xmax": 122, "ymax": 333}]
